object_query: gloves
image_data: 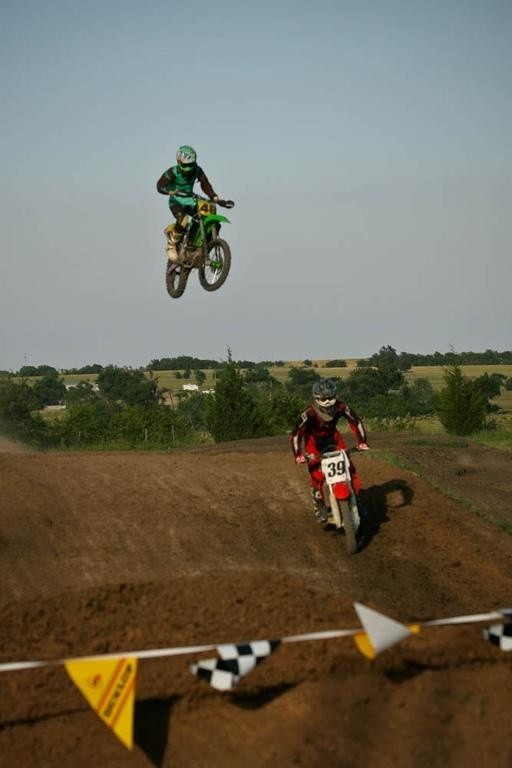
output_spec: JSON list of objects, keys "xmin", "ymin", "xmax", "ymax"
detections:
[
  {"xmin": 358, "ymin": 442, "xmax": 368, "ymax": 451},
  {"xmin": 295, "ymin": 455, "xmax": 306, "ymax": 464}
]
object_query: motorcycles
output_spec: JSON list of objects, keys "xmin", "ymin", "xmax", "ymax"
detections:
[
  {"xmin": 293, "ymin": 443, "xmax": 377, "ymax": 558},
  {"xmin": 161, "ymin": 189, "xmax": 240, "ymax": 298}
]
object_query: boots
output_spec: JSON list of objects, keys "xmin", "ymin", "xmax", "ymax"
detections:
[
  {"xmin": 311, "ymin": 488, "xmax": 327, "ymax": 522},
  {"xmin": 167, "ymin": 229, "xmax": 183, "ymax": 261},
  {"xmin": 357, "ymin": 496, "xmax": 368, "ymax": 515}
]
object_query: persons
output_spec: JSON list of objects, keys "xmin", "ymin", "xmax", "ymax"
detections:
[
  {"xmin": 157, "ymin": 145, "xmax": 220, "ymax": 262},
  {"xmin": 290, "ymin": 377, "xmax": 367, "ymax": 524}
]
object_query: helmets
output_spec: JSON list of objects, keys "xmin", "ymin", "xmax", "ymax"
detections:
[
  {"xmin": 313, "ymin": 378, "xmax": 338, "ymax": 408},
  {"xmin": 176, "ymin": 145, "xmax": 196, "ymax": 172}
]
